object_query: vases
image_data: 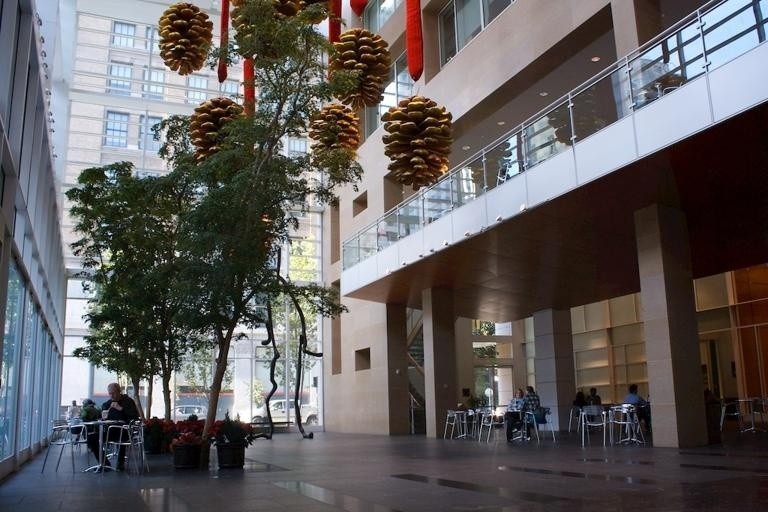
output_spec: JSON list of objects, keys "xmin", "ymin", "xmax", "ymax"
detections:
[
  {"xmin": 171, "ymin": 443, "xmax": 202, "ymax": 469},
  {"xmin": 216, "ymin": 443, "xmax": 245, "ymax": 471}
]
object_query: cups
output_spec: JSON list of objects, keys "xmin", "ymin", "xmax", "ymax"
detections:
[{"xmin": 101, "ymin": 410, "xmax": 109, "ymax": 420}]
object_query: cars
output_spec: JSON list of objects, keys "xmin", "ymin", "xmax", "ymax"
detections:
[{"xmin": 171, "ymin": 403, "xmax": 208, "ymax": 422}]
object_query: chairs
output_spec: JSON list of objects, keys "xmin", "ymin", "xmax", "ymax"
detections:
[
  {"xmin": 496, "ymin": 158, "xmax": 520, "ymax": 185},
  {"xmin": 443, "ymin": 399, "xmax": 650, "ymax": 448},
  {"xmin": 42, "ymin": 417, "xmax": 150, "ymax": 475},
  {"xmin": 720, "ymin": 396, "xmax": 768, "ymax": 435}
]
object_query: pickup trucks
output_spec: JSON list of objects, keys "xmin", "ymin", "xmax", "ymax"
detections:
[{"xmin": 252, "ymin": 398, "xmax": 320, "ymax": 427}]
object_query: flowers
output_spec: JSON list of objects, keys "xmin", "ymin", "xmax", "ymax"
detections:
[{"xmin": 143, "ymin": 414, "xmax": 256, "ymax": 448}]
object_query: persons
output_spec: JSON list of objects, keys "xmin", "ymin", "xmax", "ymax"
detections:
[
  {"xmin": 519, "ymin": 385, "xmax": 540, "ymax": 442},
  {"xmin": 87, "ymin": 383, "xmax": 140, "ymax": 473},
  {"xmin": 80, "ymin": 398, "xmax": 102, "ymax": 422},
  {"xmin": 499, "ymin": 388, "xmax": 524, "ymax": 442},
  {"xmin": 624, "ymin": 384, "xmax": 650, "ymax": 430},
  {"xmin": 572, "ymin": 392, "xmax": 591, "ymax": 430},
  {"xmin": 68, "ymin": 400, "xmax": 81, "ymax": 445},
  {"xmin": 587, "ymin": 388, "xmax": 601, "ymax": 406}
]
